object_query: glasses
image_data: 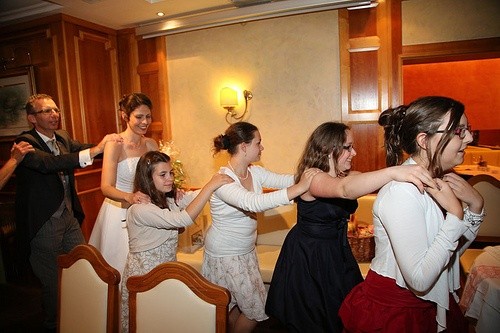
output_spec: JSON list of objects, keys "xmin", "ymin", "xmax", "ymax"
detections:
[
  {"xmin": 333, "ymin": 145, "xmax": 353, "ymax": 152},
  {"xmin": 418, "ymin": 125, "xmax": 471, "ymax": 148},
  {"xmin": 30, "ymin": 108, "xmax": 62, "ymax": 114}
]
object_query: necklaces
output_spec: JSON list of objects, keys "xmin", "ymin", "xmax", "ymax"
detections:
[{"xmin": 227, "ymin": 161, "xmax": 248, "ymax": 179}]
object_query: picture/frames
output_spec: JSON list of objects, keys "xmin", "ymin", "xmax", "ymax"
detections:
[{"xmin": 0, "ymin": 64, "xmax": 37, "ymax": 142}]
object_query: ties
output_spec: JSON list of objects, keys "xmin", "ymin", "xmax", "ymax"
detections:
[{"xmin": 50, "ymin": 139, "xmax": 72, "ymax": 216}]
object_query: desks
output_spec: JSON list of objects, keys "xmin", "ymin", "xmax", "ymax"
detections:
[
  {"xmin": 452, "ymin": 165, "xmax": 500, "ymax": 182},
  {"xmin": 462, "ymin": 146, "xmax": 500, "ymax": 167},
  {"xmin": 174, "ymin": 243, "xmax": 372, "ymax": 293},
  {"xmin": 459, "ymin": 246, "xmax": 500, "ymax": 333}
]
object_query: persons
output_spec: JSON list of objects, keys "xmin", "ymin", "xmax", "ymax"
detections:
[
  {"xmin": 86, "ymin": 93, "xmax": 185, "ymax": 292},
  {"xmin": 265, "ymin": 123, "xmax": 439, "ymax": 333},
  {"xmin": 339, "ymin": 96, "xmax": 484, "ymax": 332},
  {"xmin": 120, "ymin": 151, "xmax": 235, "ymax": 333},
  {"xmin": 0, "ymin": 94, "xmax": 129, "ymax": 333},
  {"xmin": 199, "ymin": 121, "xmax": 326, "ymax": 333}
]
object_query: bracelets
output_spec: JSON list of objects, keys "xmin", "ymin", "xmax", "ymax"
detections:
[{"xmin": 463, "ymin": 207, "xmax": 487, "ymax": 226}]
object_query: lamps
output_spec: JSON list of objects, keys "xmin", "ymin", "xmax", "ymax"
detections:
[{"xmin": 219, "ymin": 86, "xmax": 253, "ymax": 125}]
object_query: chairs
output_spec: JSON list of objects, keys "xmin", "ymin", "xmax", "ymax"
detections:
[
  {"xmin": 460, "ymin": 174, "xmax": 500, "ymax": 287},
  {"xmin": 53, "ymin": 244, "xmax": 232, "ymax": 333}
]
